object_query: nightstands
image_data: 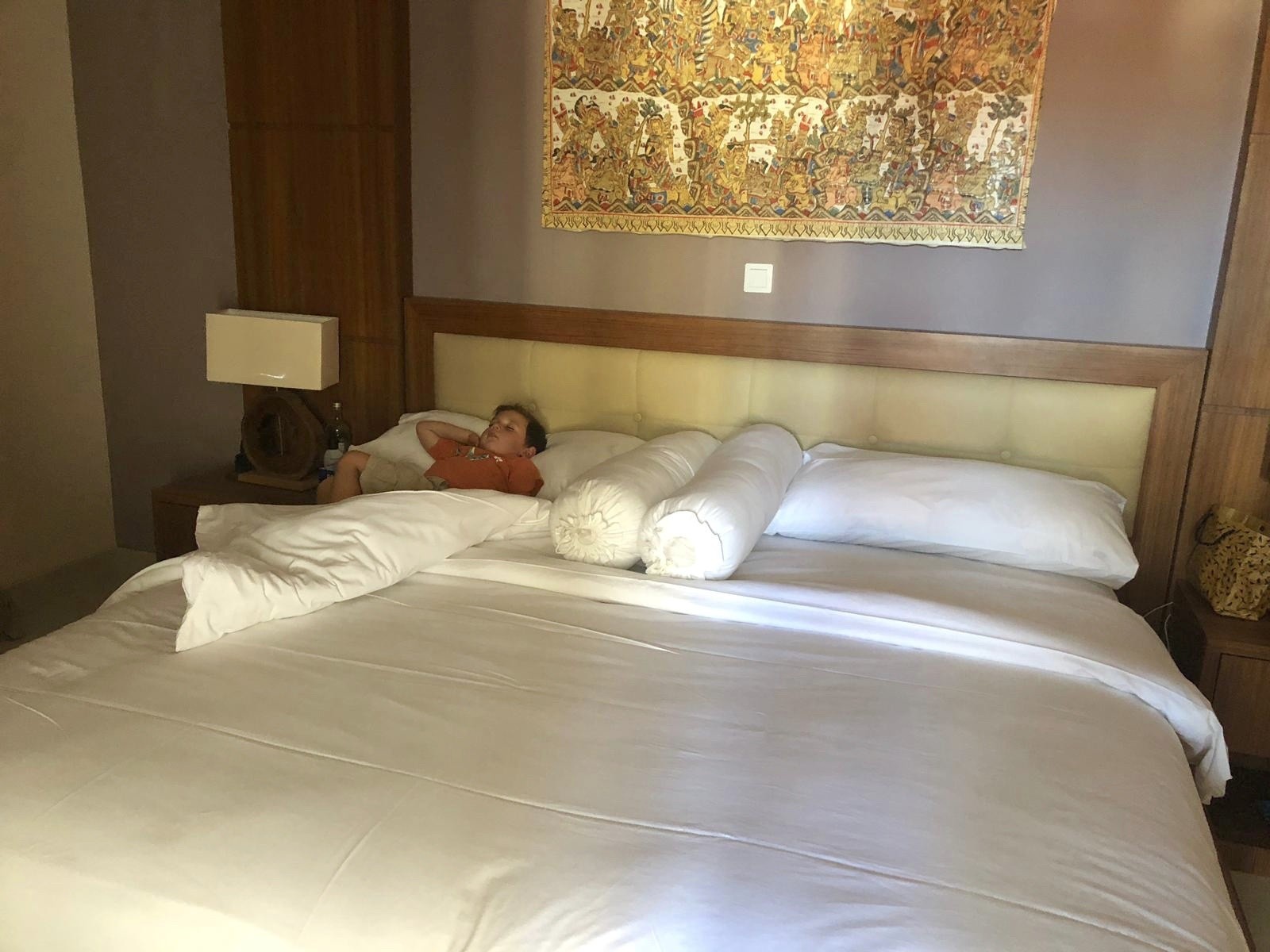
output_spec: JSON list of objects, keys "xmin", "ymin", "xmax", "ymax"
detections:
[
  {"xmin": 151, "ymin": 476, "xmax": 317, "ymax": 564},
  {"xmin": 1162, "ymin": 579, "xmax": 1270, "ymax": 770}
]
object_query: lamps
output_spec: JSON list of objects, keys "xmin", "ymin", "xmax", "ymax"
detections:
[{"xmin": 205, "ymin": 309, "xmax": 339, "ymax": 493}]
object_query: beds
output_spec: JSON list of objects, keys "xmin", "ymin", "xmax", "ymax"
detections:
[{"xmin": 0, "ymin": 295, "xmax": 1233, "ymax": 952}]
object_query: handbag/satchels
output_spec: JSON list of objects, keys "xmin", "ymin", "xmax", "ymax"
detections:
[{"xmin": 1185, "ymin": 504, "xmax": 1270, "ymax": 621}]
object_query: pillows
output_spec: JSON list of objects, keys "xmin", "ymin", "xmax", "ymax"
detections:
[{"xmin": 349, "ymin": 410, "xmax": 1141, "ymax": 590}]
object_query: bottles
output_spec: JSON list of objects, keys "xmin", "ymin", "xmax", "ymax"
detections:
[{"xmin": 324, "ymin": 401, "xmax": 350, "ymax": 453}]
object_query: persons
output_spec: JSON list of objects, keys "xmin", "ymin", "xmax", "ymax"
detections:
[{"xmin": 317, "ymin": 404, "xmax": 547, "ymax": 506}]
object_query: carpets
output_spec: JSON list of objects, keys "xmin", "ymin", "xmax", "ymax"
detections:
[
  {"xmin": 1229, "ymin": 869, "xmax": 1270, "ymax": 952},
  {"xmin": 1205, "ymin": 766, "xmax": 1270, "ymax": 849}
]
